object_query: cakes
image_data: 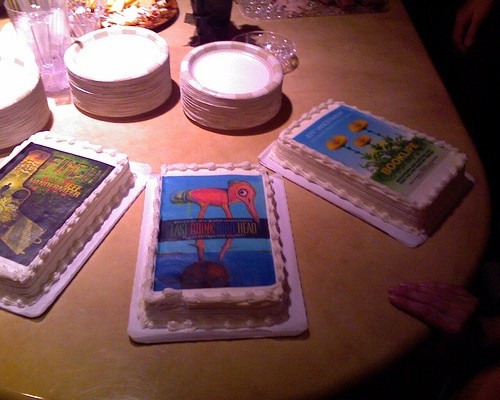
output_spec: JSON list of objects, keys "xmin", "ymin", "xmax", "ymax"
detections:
[
  {"xmin": 270, "ymin": 99, "xmax": 468, "ymax": 233},
  {"xmin": 136, "ymin": 160, "xmax": 289, "ymax": 331},
  {"xmin": 0, "ymin": 130, "xmax": 137, "ymax": 308}
]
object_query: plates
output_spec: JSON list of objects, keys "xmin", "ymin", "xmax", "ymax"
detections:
[
  {"xmin": 101, "ymin": 0, "xmax": 180, "ymax": 32},
  {"xmin": 0, "ymin": 52, "xmax": 52, "ymax": 150},
  {"xmin": 179, "ymin": 41, "xmax": 284, "ymax": 130},
  {"xmin": 63, "ymin": 25, "xmax": 172, "ymax": 118},
  {"xmin": 232, "ymin": 31, "xmax": 298, "ymax": 64}
]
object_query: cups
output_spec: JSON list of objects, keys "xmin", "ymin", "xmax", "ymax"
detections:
[
  {"xmin": 4, "ymin": 0, "xmax": 70, "ymax": 97},
  {"xmin": 64, "ymin": 1, "xmax": 102, "ymax": 37}
]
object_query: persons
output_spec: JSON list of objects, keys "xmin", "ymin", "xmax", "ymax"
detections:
[{"xmin": 386, "ymin": 282, "xmax": 500, "ymax": 400}]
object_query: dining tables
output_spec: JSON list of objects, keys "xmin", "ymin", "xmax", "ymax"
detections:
[{"xmin": 0, "ymin": 0, "xmax": 491, "ymax": 400}]
